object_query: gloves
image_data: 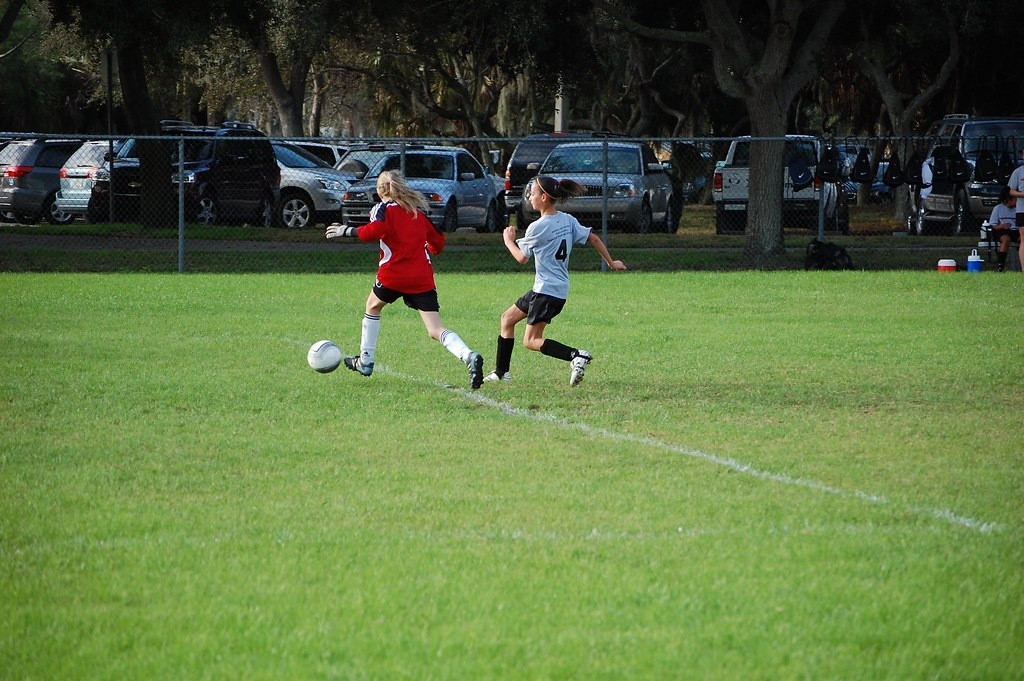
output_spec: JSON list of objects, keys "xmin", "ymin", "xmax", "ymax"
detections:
[{"xmin": 325, "ymin": 222, "xmax": 356, "ymax": 239}]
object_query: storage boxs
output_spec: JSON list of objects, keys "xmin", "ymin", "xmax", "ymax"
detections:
[{"xmin": 922, "ymin": 194, "xmax": 954, "ymax": 236}]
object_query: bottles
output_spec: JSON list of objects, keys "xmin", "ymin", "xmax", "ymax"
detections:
[{"xmin": 981, "ymin": 220, "xmax": 992, "ymax": 241}]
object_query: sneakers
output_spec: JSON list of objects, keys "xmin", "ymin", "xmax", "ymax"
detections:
[
  {"xmin": 570, "ymin": 349, "xmax": 593, "ymax": 386},
  {"xmin": 466, "ymin": 352, "xmax": 484, "ymax": 390},
  {"xmin": 482, "ymin": 370, "xmax": 512, "ymax": 383},
  {"xmin": 344, "ymin": 355, "xmax": 374, "ymax": 377},
  {"xmin": 997, "ymin": 263, "xmax": 1004, "ymax": 272}
]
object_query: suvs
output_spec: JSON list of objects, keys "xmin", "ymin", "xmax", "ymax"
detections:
[
  {"xmin": 0, "ymin": 136, "xmax": 83, "ymax": 225},
  {"xmin": 504, "ymin": 129, "xmax": 638, "ymax": 228},
  {"xmin": 268, "ymin": 140, "xmax": 362, "ymax": 228},
  {"xmin": 87, "ymin": 119, "xmax": 282, "ymax": 228},
  {"xmin": 904, "ymin": 113, "xmax": 1024, "ymax": 235},
  {"xmin": 522, "ymin": 139, "xmax": 684, "ymax": 235},
  {"xmin": 55, "ymin": 139, "xmax": 120, "ymax": 221}
]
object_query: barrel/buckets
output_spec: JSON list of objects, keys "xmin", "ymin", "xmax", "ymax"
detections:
[
  {"xmin": 938, "ymin": 259, "xmax": 956, "ymax": 271},
  {"xmin": 968, "ymin": 248, "xmax": 980, "ymax": 273}
]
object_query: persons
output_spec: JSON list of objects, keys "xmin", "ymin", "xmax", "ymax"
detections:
[
  {"xmin": 326, "ymin": 170, "xmax": 485, "ymax": 391},
  {"xmin": 482, "ymin": 176, "xmax": 627, "ymax": 387},
  {"xmin": 989, "ymin": 147, "xmax": 1024, "ymax": 274}
]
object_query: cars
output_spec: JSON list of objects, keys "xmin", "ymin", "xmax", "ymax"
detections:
[
  {"xmin": 661, "ymin": 149, "xmax": 714, "ymax": 203},
  {"xmin": 335, "ymin": 141, "xmax": 510, "ymax": 233},
  {"xmin": 839, "ymin": 144, "xmax": 898, "ymax": 205}
]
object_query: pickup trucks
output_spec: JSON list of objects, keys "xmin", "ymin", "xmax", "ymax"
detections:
[{"xmin": 713, "ymin": 133, "xmax": 846, "ymax": 233}]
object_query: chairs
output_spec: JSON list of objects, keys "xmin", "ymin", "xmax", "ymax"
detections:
[{"xmin": 613, "ymin": 154, "xmax": 637, "ymax": 174}]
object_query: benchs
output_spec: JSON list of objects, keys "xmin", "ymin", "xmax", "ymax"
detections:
[{"xmin": 977, "ymin": 241, "xmax": 1020, "ymax": 271}]
